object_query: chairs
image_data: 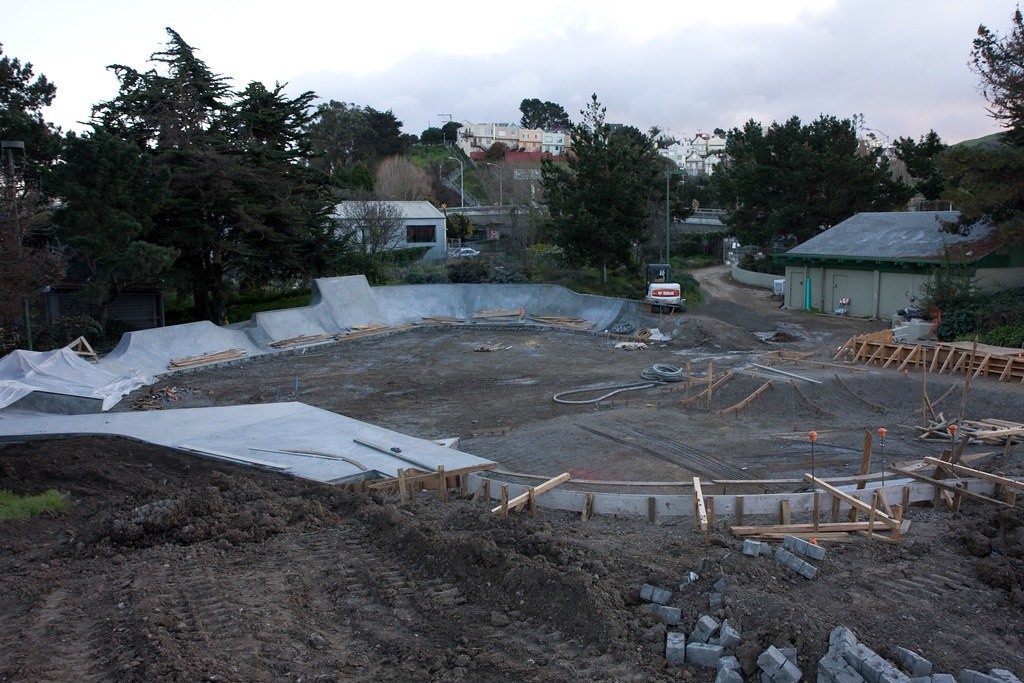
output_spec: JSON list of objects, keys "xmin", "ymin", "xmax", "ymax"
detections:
[{"xmin": 834, "ymin": 297, "xmax": 850, "ymax": 317}]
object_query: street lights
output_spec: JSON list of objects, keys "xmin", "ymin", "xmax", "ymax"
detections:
[
  {"xmin": 448, "ymin": 157, "xmax": 463, "ymax": 208},
  {"xmin": 486, "ymin": 162, "xmax": 503, "ymax": 205},
  {"xmin": 663, "ymin": 163, "xmax": 689, "ymax": 283}
]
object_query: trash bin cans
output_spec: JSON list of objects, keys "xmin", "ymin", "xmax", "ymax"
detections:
[{"xmin": 774, "ymin": 279, "xmax": 785, "ymax": 296}]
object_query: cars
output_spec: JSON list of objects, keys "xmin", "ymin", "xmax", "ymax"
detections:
[{"xmin": 448, "ymin": 247, "xmax": 480, "ymax": 259}]
